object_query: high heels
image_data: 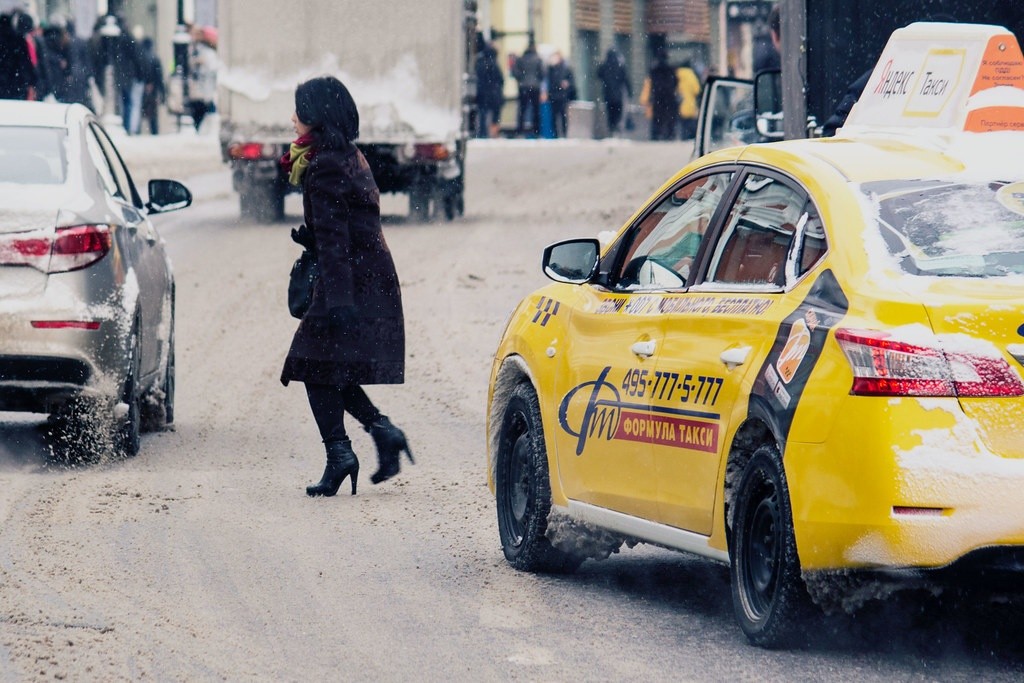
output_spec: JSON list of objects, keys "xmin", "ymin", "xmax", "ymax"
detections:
[
  {"xmin": 364, "ymin": 413, "xmax": 417, "ymax": 484},
  {"xmin": 306, "ymin": 439, "xmax": 359, "ymax": 501}
]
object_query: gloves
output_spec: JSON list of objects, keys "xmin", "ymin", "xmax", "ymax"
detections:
[{"xmin": 291, "ymin": 225, "xmax": 309, "ymax": 246}]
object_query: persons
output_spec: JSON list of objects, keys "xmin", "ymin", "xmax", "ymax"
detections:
[
  {"xmin": 280, "ymin": 75, "xmax": 415, "ymax": 497},
  {"xmin": 470, "ymin": 2, "xmax": 784, "ymax": 142},
  {"xmin": 0, "ymin": 7, "xmax": 218, "ymax": 136}
]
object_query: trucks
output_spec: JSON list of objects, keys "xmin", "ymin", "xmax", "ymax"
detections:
[{"xmin": 215, "ymin": 0, "xmax": 480, "ymax": 219}]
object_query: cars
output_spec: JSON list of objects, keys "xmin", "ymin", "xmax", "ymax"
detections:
[
  {"xmin": 487, "ymin": 20, "xmax": 1024, "ymax": 650},
  {"xmin": 0, "ymin": 100, "xmax": 191, "ymax": 455}
]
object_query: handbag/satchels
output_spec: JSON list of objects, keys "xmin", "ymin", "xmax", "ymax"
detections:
[
  {"xmin": 624, "ymin": 113, "xmax": 636, "ymax": 131},
  {"xmin": 288, "ymin": 250, "xmax": 315, "ymax": 318}
]
